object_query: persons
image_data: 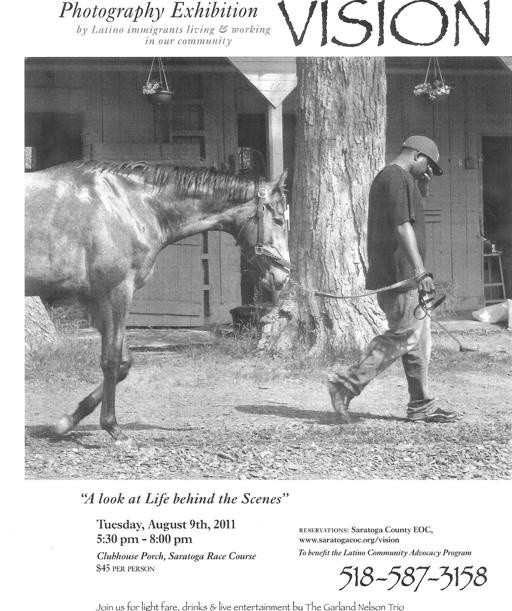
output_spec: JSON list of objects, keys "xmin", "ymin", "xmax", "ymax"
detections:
[{"xmin": 327, "ymin": 134, "xmax": 460, "ymax": 424}]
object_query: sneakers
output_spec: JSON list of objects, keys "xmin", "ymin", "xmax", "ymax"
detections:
[
  {"xmin": 328, "ymin": 381, "xmax": 355, "ymax": 424},
  {"xmin": 406, "ymin": 406, "xmax": 458, "ymax": 422}
]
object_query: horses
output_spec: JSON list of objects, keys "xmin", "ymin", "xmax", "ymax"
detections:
[{"xmin": 25, "ymin": 155, "xmax": 294, "ymax": 451}]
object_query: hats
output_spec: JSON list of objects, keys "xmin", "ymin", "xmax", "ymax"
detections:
[{"xmin": 400, "ymin": 134, "xmax": 443, "ymax": 177}]
